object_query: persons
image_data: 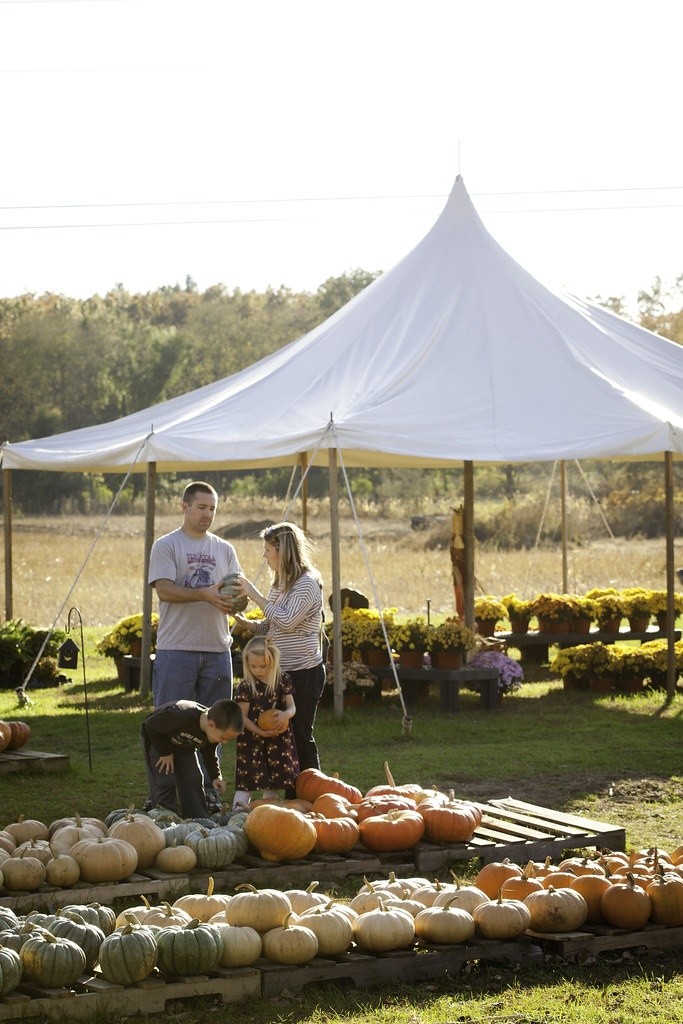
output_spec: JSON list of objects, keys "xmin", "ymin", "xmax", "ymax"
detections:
[
  {"xmin": 148, "ymin": 482, "xmax": 243, "ymax": 710},
  {"xmin": 140, "ymin": 699, "xmax": 243, "ymax": 818},
  {"xmin": 233, "ymin": 634, "xmax": 300, "ymax": 809},
  {"xmin": 236, "ymin": 524, "xmax": 326, "ymax": 798}
]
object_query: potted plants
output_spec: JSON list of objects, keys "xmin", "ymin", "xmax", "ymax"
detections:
[
  {"xmin": 94, "ymin": 606, "xmax": 471, "ymax": 668},
  {"xmin": 472, "ymin": 587, "xmax": 683, "ymax": 695}
]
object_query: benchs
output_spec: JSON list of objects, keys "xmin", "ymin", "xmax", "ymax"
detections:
[{"xmin": 121, "ymin": 653, "xmax": 156, "ymax": 692}]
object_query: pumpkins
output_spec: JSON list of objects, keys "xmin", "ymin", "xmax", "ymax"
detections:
[
  {"xmin": 258, "ymin": 702, "xmax": 288, "ymax": 734},
  {"xmin": 220, "ymin": 571, "xmax": 248, "ymax": 613},
  {"xmin": 0, "ymin": 761, "xmax": 683, "ymax": 998},
  {"xmin": 0, "ymin": 720, "xmax": 31, "ymax": 752}
]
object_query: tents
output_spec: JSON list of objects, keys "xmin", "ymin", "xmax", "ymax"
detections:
[{"xmin": 0, "ymin": 176, "xmax": 683, "ymax": 692}]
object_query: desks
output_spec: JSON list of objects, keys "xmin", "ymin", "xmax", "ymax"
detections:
[
  {"xmin": 489, "ymin": 626, "xmax": 681, "ymax": 659},
  {"xmin": 353, "ymin": 665, "xmax": 501, "ymax": 714}
]
